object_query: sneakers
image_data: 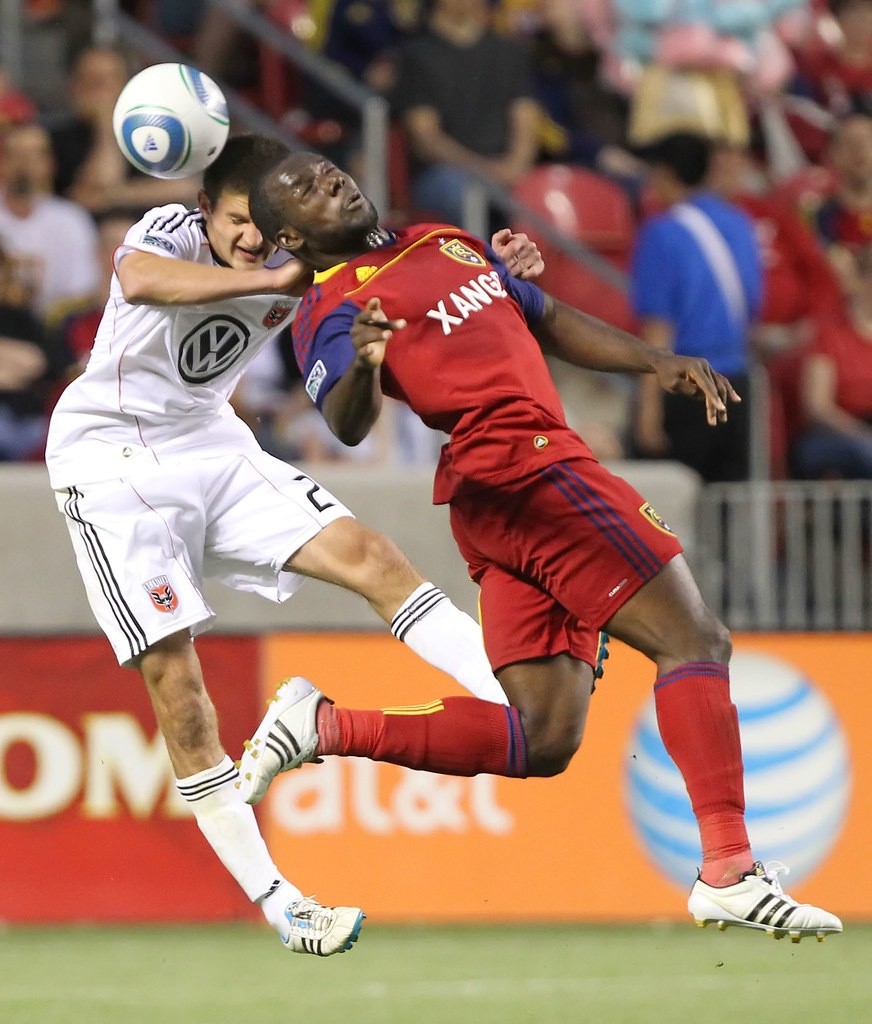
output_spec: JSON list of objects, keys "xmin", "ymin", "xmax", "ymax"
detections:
[
  {"xmin": 279, "ymin": 895, "xmax": 366, "ymax": 956},
  {"xmin": 234, "ymin": 674, "xmax": 336, "ymax": 804},
  {"xmin": 687, "ymin": 861, "xmax": 843, "ymax": 941},
  {"xmin": 589, "ymin": 629, "xmax": 611, "ymax": 693}
]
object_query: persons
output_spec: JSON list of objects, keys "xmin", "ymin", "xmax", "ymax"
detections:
[
  {"xmin": 45, "ymin": 134, "xmax": 610, "ymax": 955},
  {"xmin": 1, "ymin": 0, "xmax": 872, "ymax": 626},
  {"xmin": 240, "ymin": 148, "xmax": 845, "ymax": 946}
]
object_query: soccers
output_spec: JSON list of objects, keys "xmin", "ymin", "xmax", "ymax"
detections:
[{"xmin": 110, "ymin": 59, "xmax": 234, "ymax": 185}]
